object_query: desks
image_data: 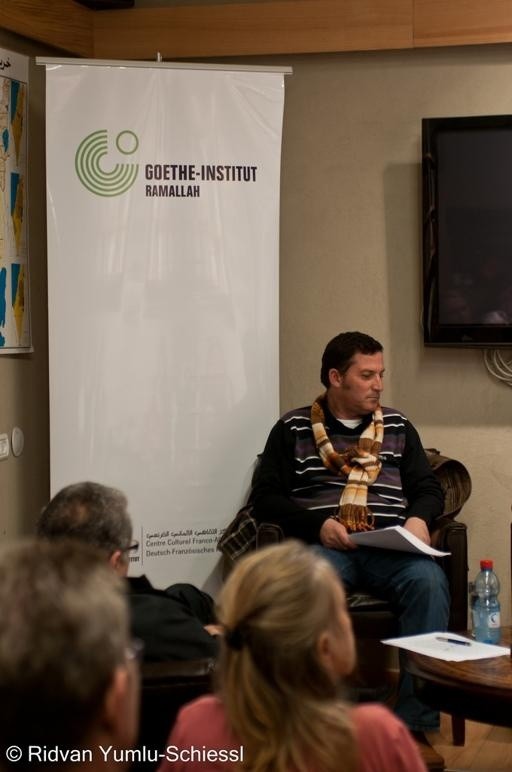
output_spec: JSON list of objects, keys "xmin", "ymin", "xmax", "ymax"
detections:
[{"xmin": 399, "ymin": 625, "xmax": 511, "ymax": 730}]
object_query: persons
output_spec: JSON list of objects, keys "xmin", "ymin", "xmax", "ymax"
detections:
[
  {"xmin": 160, "ymin": 539, "xmax": 430, "ymax": 772},
  {"xmin": 0, "ymin": 538, "xmax": 142, "ymax": 772},
  {"xmin": 260, "ymin": 331, "xmax": 452, "ymax": 772},
  {"xmin": 37, "ymin": 479, "xmax": 222, "ymax": 661}
]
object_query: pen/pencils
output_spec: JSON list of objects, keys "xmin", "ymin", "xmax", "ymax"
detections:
[{"xmin": 436, "ymin": 635, "xmax": 471, "ymax": 647}]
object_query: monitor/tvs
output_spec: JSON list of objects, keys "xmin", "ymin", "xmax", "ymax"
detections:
[{"xmin": 422, "ymin": 114, "xmax": 512, "ymax": 348}]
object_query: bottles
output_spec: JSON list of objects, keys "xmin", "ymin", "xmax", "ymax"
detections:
[{"xmin": 473, "ymin": 559, "xmax": 502, "ymax": 645}]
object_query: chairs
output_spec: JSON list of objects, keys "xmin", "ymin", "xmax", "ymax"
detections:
[
  {"xmin": 144, "ymin": 657, "xmax": 212, "ymax": 704},
  {"xmin": 259, "ymin": 522, "xmax": 468, "ymax": 746}
]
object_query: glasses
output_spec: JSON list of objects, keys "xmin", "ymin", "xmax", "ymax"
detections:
[{"xmin": 122, "ymin": 540, "xmax": 138, "ymax": 554}]
